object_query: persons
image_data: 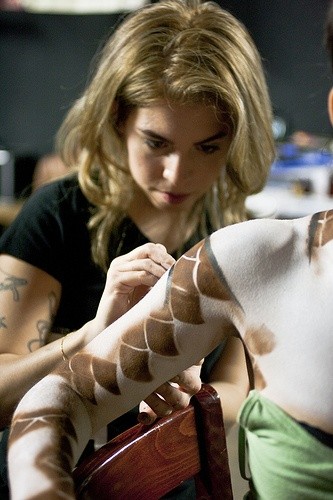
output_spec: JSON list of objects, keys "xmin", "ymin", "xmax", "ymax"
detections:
[
  {"xmin": 0, "ymin": 0, "xmax": 277, "ymax": 500},
  {"xmin": 7, "ymin": 7, "xmax": 333, "ymax": 499}
]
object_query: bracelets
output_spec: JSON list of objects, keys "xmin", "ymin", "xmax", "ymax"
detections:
[{"xmin": 61, "ymin": 336, "xmax": 69, "ymax": 360}]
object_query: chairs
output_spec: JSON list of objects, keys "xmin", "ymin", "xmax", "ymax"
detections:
[{"xmin": 73, "ymin": 384, "xmax": 234, "ymax": 500}]
